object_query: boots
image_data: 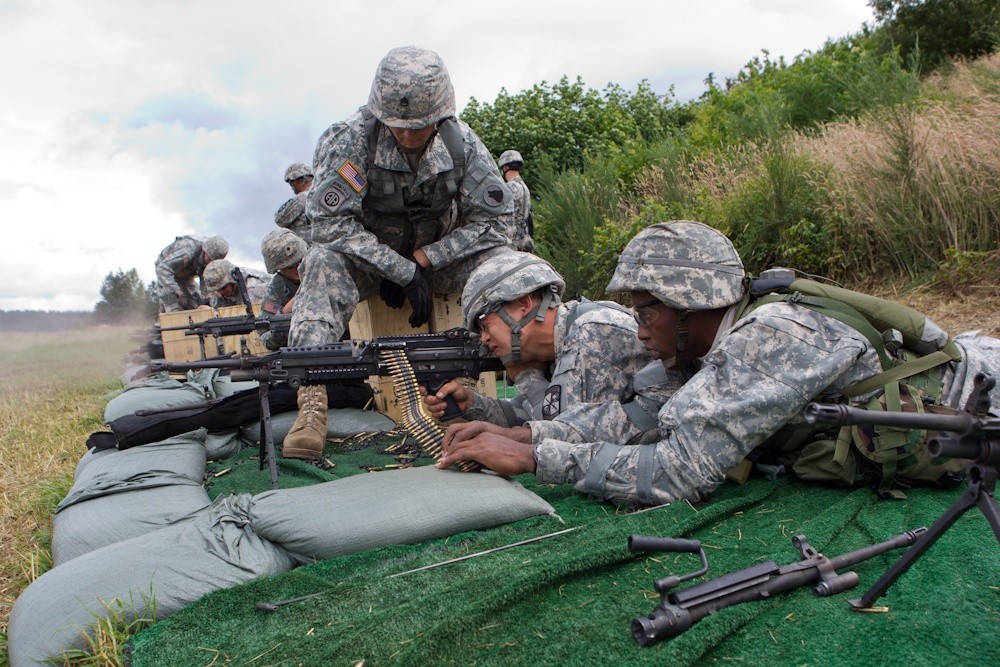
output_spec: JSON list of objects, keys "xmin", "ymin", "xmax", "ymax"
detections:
[{"xmin": 282, "ymin": 384, "xmax": 329, "ymax": 460}]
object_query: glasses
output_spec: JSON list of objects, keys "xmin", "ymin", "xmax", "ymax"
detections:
[{"xmin": 634, "ymin": 307, "xmax": 660, "ymax": 328}]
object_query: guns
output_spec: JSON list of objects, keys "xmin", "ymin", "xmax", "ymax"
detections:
[
  {"xmin": 148, "ymin": 267, "xmax": 294, "ymax": 362},
  {"xmin": 802, "ymin": 398, "xmax": 1000, "ymax": 616},
  {"xmin": 128, "ymin": 321, "xmax": 163, "ymax": 358},
  {"xmin": 148, "ymin": 327, "xmax": 505, "ymax": 491}
]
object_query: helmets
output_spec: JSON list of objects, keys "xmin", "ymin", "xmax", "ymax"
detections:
[
  {"xmin": 605, "ymin": 219, "xmax": 745, "ymax": 309},
  {"xmin": 202, "ymin": 259, "xmax": 237, "ymax": 291},
  {"xmin": 498, "ymin": 150, "xmax": 524, "ymax": 169},
  {"xmin": 201, "ymin": 235, "xmax": 229, "ymax": 261},
  {"xmin": 461, "ymin": 252, "xmax": 566, "ymax": 333},
  {"xmin": 367, "ymin": 46, "xmax": 456, "ymax": 130},
  {"xmin": 284, "ymin": 162, "xmax": 314, "ymax": 182},
  {"xmin": 274, "ymin": 196, "xmax": 307, "ymax": 240},
  {"xmin": 261, "ymin": 227, "xmax": 309, "ymax": 274}
]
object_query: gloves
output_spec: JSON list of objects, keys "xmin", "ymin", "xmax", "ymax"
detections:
[
  {"xmin": 378, "ymin": 254, "xmax": 424, "ymax": 310},
  {"xmin": 403, "ymin": 267, "xmax": 434, "ymax": 328}
]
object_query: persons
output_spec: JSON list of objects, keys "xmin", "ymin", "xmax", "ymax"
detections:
[
  {"xmin": 280, "ymin": 47, "xmax": 517, "ymax": 459},
  {"xmin": 146, "ymin": 148, "xmax": 1000, "ymax": 503}
]
